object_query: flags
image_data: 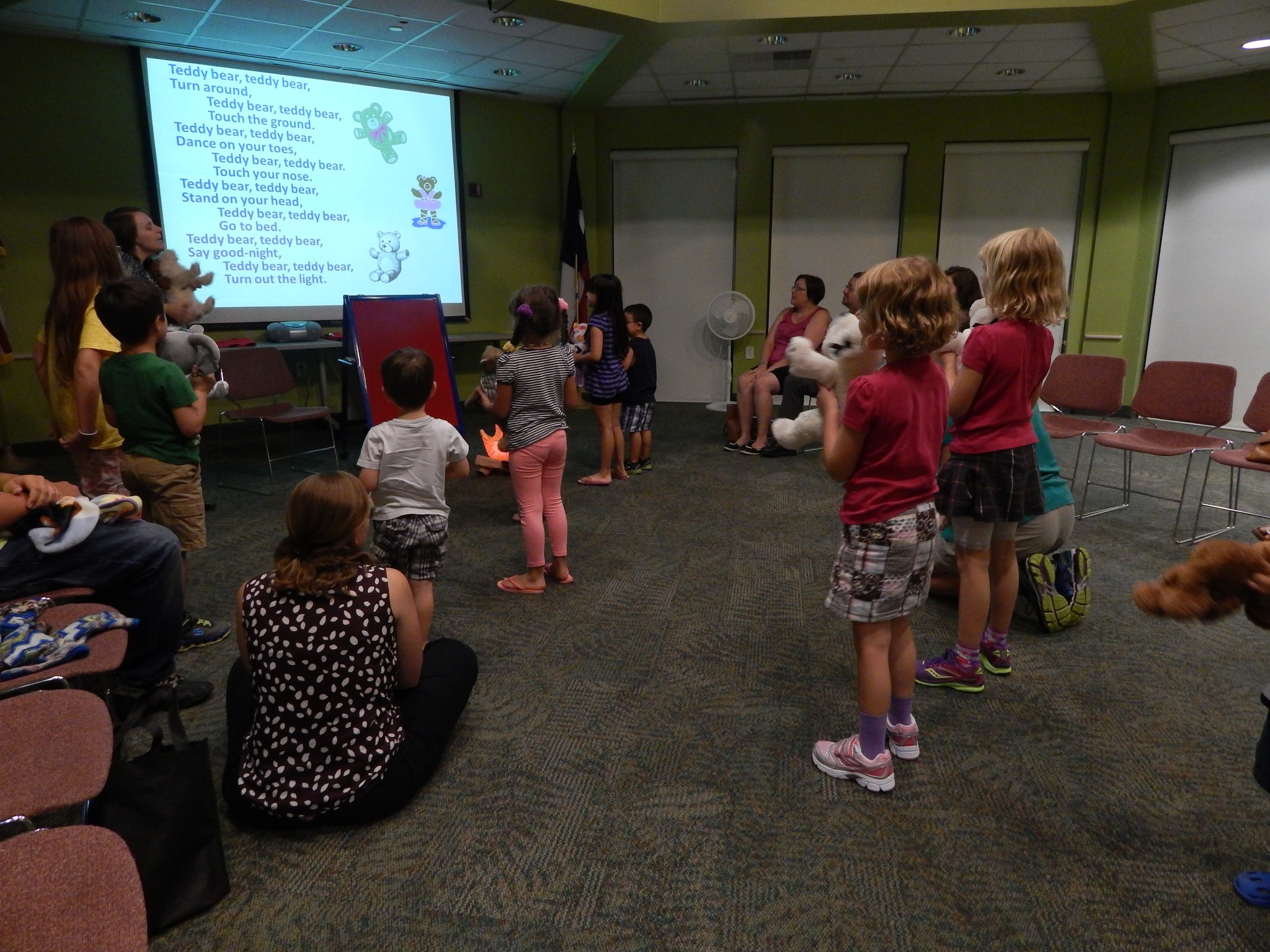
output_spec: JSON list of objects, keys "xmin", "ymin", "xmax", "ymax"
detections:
[{"xmin": 559, "ymin": 152, "xmax": 590, "ymax": 332}]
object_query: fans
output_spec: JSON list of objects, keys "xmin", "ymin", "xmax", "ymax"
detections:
[{"xmin": 705, "ymin": 292, "xmax": 755, "ymax": 410}]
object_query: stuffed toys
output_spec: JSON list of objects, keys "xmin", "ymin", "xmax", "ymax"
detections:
[
  {"xmin": 771, "ymin": 313, "xmax": 886, "ymax": 451},
  {"xmin": 465, "ymin": 345, "xmax": 504, "ymax": 411},
  {"xmin": 933, "ymin": 298, "xmax": 1000, "ymax": 374},
  {"xmin": 156, "ymin": 323, "xmax": 228, "ymax": 401},
  {"xmin": 146, "ymin": 248, "xmax": 214, "ymax": 323},
  {"xmin": 1130, "ymin": 537, "xmax": 1270, "ymax": 627},
  {"xmin": 559, "ymin": 322, "xmax": 588, "ymax": 357}
]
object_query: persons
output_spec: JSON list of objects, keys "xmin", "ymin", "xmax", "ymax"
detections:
[
  {"xmin": 220, "ymin": 470, "xmax": 478, "ymax": 833},
  {"xmin": 813, "ymin": 257, "xmax": 959, "ymax": 790},
  {"xmin": 475, "ymin": 284, "xmax": 580, "ymax": 594},
  {"xmin": 0, "ymin": 206, "xmax": 233, "ymax": 709},
  {"xmin": 1232, "ymin": 522, "xmax": 1270, "ymax": 907},
  {"xmin": 356, "ymin": 348, "xmax": 470, "ymax": 652},
  {"xmin": 723, "ymin": 226, "xmax": 1091, "ymax": 693},
  {"xmin": 573, "ymin": 274, "xmax": 658, "ymax": 484}
]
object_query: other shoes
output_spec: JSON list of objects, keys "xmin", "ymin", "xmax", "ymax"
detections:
[
  {"xmin": 724, "ymin": 440, "xmax": 753, "ymax": 451},
  {"xmin": 761, "ymin": 441, "xmax": 797, "ymax": 457},
  {"xmin": 742, "ymin": 442, "xmax": 770, "ymax": 454}
]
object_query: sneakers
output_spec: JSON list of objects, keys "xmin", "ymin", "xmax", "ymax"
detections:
[
  {"xmin": 915, "ymin": 645, "xmax": 984, "ymax": 692},
  {"xmin": 884, "ymin": 710, "xmax": 920, "ymax": 759},
  {"xmin": 1014, "ymin": 553, "xmax": 1071, "ymax": 633},
  {"xmin": 148, "ymin": 672, "xmax": 214, "ymax": 712},
  {"xmin": 638, "ymin": 457, "xmax": 652, "ymax": 469},
  {"xmin": 977, "ymin": 636, "xmax": 1013, "ymax": 673},
  {"xmin": 624, "ymin": 462, "xmax": 642, "ymax": 474},
  {"xmin": 1052, "ymin": 547, "xmax": 1090, "ymax": 626},
  {"xmin": 175, "ymin": 610, "xmax": 232, "ymax": 652},
  {"xmin": 812, "ymin": 732, "xmax": 896, "ymax": 791}
]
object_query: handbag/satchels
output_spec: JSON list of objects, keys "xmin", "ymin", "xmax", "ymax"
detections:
[{"xmin": 85, "ymin": 705, "xmax": 231, "ymax": 937}]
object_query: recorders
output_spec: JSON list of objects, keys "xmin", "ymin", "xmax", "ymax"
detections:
[{"xmin": 265, "ymin": 319, "xmax": 323, "ymax": 344}]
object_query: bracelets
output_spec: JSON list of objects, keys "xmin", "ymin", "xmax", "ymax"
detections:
[{"xmin": 78, "ymin": 429, "xmax": 98, "ymax": 436}]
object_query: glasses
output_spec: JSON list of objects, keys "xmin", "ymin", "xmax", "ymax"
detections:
[{"xmin": 791, "ymin": 286, "xmax": 807, "ymax": 293}]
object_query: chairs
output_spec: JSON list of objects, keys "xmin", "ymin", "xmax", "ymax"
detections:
[
  {"xmin": 0, "ymin": 589, "xmax": 150, "ymax": 952},
  {"xmin": 211, "ymin": 348, "xmax": 342, "ymax": 495},
  {"xmin": 1037, "ymin": 354, "xmax": 1270, "ymax": 545}
]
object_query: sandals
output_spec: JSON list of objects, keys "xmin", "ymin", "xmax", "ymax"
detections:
[{"xmin": 1232, "ymin": 872, "xmax": 1270, "ymax": 908}]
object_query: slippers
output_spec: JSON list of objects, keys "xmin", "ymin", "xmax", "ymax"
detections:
[
  {"xmin": 610, "ymin": 473, "xmax": 629, "ymax": 479},
  {"xmin": 544, "ymin": 563, "xmax": 573, "ymax": 584},
  {"xmin": 497, "ymin": 575, "xmax": 544, "ymax": 594},
  {"xmin": 577, "ymin": 476, "xmax": 610, "ymax": 485}
]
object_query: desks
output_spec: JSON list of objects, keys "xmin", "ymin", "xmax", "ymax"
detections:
[{"xmin": 223, "ymin": 331, "xmax": 513, "ymax": 434}]
